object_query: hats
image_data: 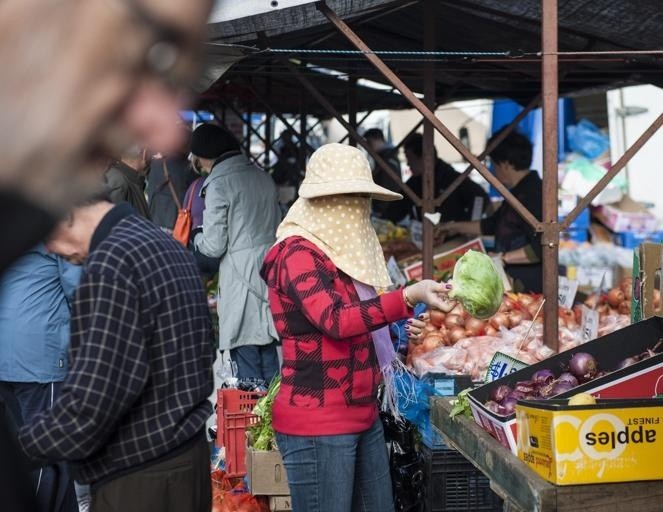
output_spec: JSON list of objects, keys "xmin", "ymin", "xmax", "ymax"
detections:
[
  {"xmin": 298, "ymin": 143, "xmax": 404, "ymax": 202},
  {"xmin": 192, "ymin": 124, "xmax": 240, "ymax": 158}
]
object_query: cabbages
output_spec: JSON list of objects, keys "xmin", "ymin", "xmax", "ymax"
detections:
[{"xmin": 448, "ymin": 249, "xmax": 504, "ymax": 319}]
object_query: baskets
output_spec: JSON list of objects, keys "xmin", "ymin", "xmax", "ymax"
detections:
[
  {"xmin": 217, "ymin": 388, "xmax": 267, "ymax": 477},
  {"xmin": 420, "ymin": 434, "xmax": 504, "ymax": 512}
]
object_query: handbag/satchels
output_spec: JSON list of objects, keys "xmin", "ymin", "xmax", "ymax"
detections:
[
  {"xmin": 173, "ymin": 178, "xmax": 199, "ymax": 247},
  {"xmin": 380, "ymin": 412, "xmax": 427, "ymax": 512}
]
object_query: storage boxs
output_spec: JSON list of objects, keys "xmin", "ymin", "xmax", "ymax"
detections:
[
  {"xmin": 465, "ymin": 317, "xmax": 660, "ymax": 485},
  {"xmin": 399, "ymin": 235, "xmax": 487, "ymax": 285},
  {"xmin": 245, "ymin": 449, "xmax": 292, "ymax": 511},
  {"xmin": 556, "ymin": 157, "xmax": 661, "ymax": 250}
]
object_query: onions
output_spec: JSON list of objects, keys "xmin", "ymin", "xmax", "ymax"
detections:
[{"xmin": 406, "ymin": 278, "xmax": 661, "ymax": 415}]
